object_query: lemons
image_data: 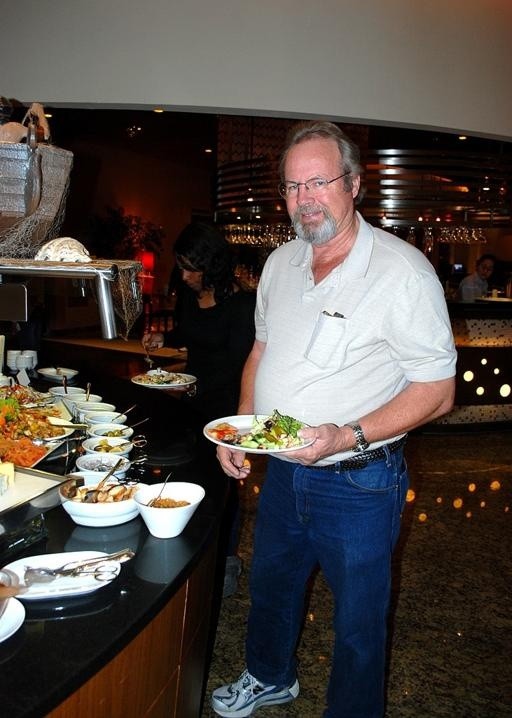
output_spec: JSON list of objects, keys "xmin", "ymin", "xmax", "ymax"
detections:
[{"xmin": 109, "ymin": 447, "xmax": 123, "ymax": 452}]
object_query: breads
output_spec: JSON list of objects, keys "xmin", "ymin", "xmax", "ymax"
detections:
[
  {"xmin": 0, "ymin": 460, "xmax": 15, "ymax": 492},
  {"xmin": 74, "ymin": 483, "xmax": 134, "ymax": 502}
]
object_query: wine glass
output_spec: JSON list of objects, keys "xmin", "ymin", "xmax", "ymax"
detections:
[
  {"xmin": 388, "ymin": 227, "xmax": 489, "ymax": 251},
  {"xmin": 221, "ymin": 225, "xmax": 300, "ymax": 250}
]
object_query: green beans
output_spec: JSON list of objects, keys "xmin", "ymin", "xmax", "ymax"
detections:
[{"xmin": 150, "ymin": 499, "xmax": 190, "ymax": 509}]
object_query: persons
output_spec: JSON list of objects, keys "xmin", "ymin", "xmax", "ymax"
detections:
[
  {"xmin": 140, "ymin": 221, "xmax": 257, "ymax": 600},
  {"xmin": 458, "ymin": 253, "xmax": 498, "ymax": 301},
  {"xmin": 211, "ymin": 122, "xmax": 461, "ymax": 717}
]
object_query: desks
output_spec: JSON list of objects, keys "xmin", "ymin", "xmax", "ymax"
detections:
[{"xmin": 47, "ymin": 332, "xmax": 184, "ymax": 357}]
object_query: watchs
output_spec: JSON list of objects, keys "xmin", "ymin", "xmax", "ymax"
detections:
[{"xmin": 344, "ymin": 422, "xmax": 369, "ymax": 452}]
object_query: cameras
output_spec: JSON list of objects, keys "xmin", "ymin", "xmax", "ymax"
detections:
[{"xmin": 454, "ymin": 263, "xmax": 463, "ymax": 272}]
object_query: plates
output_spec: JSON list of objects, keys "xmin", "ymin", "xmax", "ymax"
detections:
[
  {"xmin": 129, "ymin": 372, "xmax": 196, "ymax": 390},
  {"xmin": 43, "ymin": 386, "xmax": 135, "ymax": 475},
  {"xmin": 204, "ymin": 412, "xmax": 318, "ymax": 453},
  {"xmin": 37, "ymin": 367, "xmax": 78, "ymax": 380},
  {"xmin": 0, "ymin": 595, "xmax": 25, "ymax": 642},
  {"xmin": 2, "ymin": 549, "xmax": 118, "ymax": 600}
]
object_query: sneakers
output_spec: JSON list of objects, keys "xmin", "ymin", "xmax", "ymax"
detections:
[{"xmin": 211, "ymin": 669, "xmax": 300, "ymax": 717}]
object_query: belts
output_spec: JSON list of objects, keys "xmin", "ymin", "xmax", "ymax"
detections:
[{"xmin": 297, "ymin": 435, "xmax": 409, "ymax": 471}]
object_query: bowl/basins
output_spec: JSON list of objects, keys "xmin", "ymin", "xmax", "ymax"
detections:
[
  {"xmin": 134, "ymin": 481, "xmax": 206, "ymax": 540},
  {"xmin": 65, "ymin": 518, "xmax": 143, "ymax": 559},
  {"xmin": 30, "ymin": 486, "xmax": 60, "ymax": 509},
  {"xmin": 133, "ymin": 535, "xmax": 189, "ymax": 586},
  {"xmin": 58, "ymin": 480, "xmax": 146, "ymax": 528},
  {"xmin": 7, "ymin": 349, "xmax": 39, "ymax": 371}
]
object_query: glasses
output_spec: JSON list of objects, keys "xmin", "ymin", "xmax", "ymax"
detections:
[{"xmin": 278, "ymin": 170, "xmax": 353, "ymax": 199}]
